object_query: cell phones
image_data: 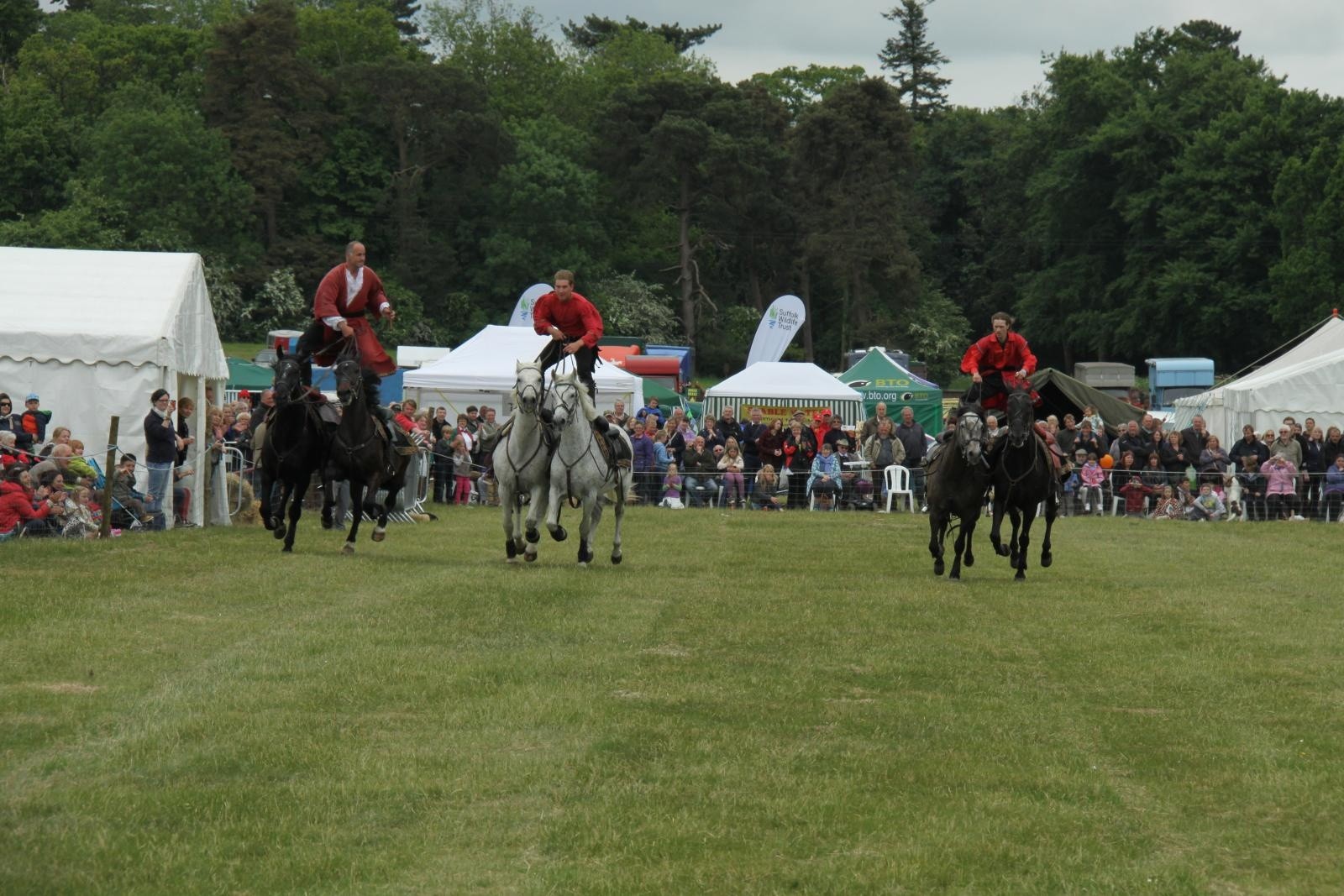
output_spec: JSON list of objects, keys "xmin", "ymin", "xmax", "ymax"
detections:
[{"xmin": 171, "ymin": 401, "xmax": 176, "ymax": 411}]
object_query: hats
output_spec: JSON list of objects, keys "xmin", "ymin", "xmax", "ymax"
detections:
[
  {"xmin": 1075, "ymin": 449, "xmax": 1088, "ymax": 455},
  {"xmin": 392, "ymin": 404, "xmax": 401, "ymax": 410},
  {"xmin": 835, "ymin": 439, "xmax": 849, "ymax": 446},
  {"xmin": 814, "ymin": 414, "xmax": 822, "ymax": 421},
  {"xmin": 793, "ymin": 410, "xmax": 805, "ymax": 417},
  {"xmin": 26, "ymin": 394, "xmax": 39, "ymax": 402},
  {"xmin": 823, "ymin": 410, "xmax": 832, "ymax": 416},
  {"xmin": 604, "ymin": 410, "xmax": 612, "ymax": 418}
]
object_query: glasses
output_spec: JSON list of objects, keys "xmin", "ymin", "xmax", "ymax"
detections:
[
  {"xmin": 1266, "ymin": 434, "xmax": 1274, "ymax": 437},
  {"xmin": 0, "ymin": 402, "xmax": 11, "ymax": 407},
  {"xmin": 1279, "ymin": 431, "xmax": 1288, "ymax": 434},
  {"xmin": 158, "ymin": 399, "xmax": 169, "ymax": 402},
  {"xmin": 1118, "ymin": 430, "xmax": 1126, "ymax": 432}
]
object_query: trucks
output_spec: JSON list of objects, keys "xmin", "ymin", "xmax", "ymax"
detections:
[
  {"xmin": 1146, "ymin": 357, "xmax": 1215, "ymax": 412},
  {"xmin": 596, "ymin": 335, "xmax": 693, "ymax": 398},
  {"xmin": 1074, "ymin": 361, "xmax": 1145, "ymax": 411}
]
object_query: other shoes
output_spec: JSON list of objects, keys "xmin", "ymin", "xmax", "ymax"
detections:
[
  {"xmin": 1085, "ymin": 503, "xmax": 1090, "ymax": 511},
  {"xmin": 1097, "ymin": 503, "xmax": 1103, "ymax": 511},
  {"xmin": 175, "ymin": 519, "xmax": 195, "ymax": 528},
  {"xmin": 141, "ymin": 515, "xmax": 153, "ymax": 523}
]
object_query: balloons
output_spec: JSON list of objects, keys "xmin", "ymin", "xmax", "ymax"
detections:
[{"xmin": 1100, "ymin": 454, "xmax": 1113, "ymax": 469}]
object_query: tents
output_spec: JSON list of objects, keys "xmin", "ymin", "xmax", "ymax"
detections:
[
  {"xmin": 621, "ymin": 368, "xmax": 706, "ymax": 420},
  {"xmin": 1, "ymin": 245, "xmax": 233, "ymax": 528},
  {"xmin": 837, "ymin": 346, "xmax": 942, "ymax": 438},
  {"xmin": 312, "ymin": 345, "xmax": 451, "ymax": 408},
  {"xmin": 701, "ymin": 361, "xmax": 866, "ymax": 430},
  {"xmin": 1030, "ymin": 368, "xmax": 1147, "ymax": 437},
  {"xmin": 1173, "ymin": 309, "xmax": 1344, "ymax": 472},
  {"xmin": 226, "ymin": 358, "xmax": 276, "ymax": 405},
  {"xmin": 403, "ymin": 324, "xmax": 644, "ymax": 432}
]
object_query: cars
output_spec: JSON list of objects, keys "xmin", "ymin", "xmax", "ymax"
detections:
[{"xmin": 253, "ymin": 348, "xmax": 277, "ymax": 369}]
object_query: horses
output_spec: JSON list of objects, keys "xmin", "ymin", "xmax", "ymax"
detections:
[
  {"xmin": 926, "ymin": 398, "xmax": 991, "ymax": 581},
  {"xmin": 321, "ymin": 349, "xmax": 413, "ymax": 556},
  {"xmin": 256, "ymin": 344, "xmax": 347, "ymax": 554},
  {"xmin": 991, "ymin": 376, "xmax": 1059, "ymax": 581},
  {"xmin": 544, "ymin": 369, "xmax": 633, "ymax": 567},
  {"xmin": 492, "ymin": 355, "xmax": 555, "ymax": 563}
]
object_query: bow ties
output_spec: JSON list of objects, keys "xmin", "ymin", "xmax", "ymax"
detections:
[{"xmin": 460, "ymin": 430, "xmax": 468, "ymax": 433}]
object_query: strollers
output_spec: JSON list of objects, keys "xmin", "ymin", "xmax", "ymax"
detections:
[{"xmin": 87, "ymin": 457, "xmax": 156, "ymax": 531}]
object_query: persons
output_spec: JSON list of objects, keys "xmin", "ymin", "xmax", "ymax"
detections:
[
  {"xmin": 296, "ymin": 240, "xmax": 396, "ymax": 415},
  {"xmin": 961, "ymin": 312, "xmax": 1042, "ymax": 420},
  {"xmin": 533, "ymin": 269, "xmax": 604, "ymax": 409},
  {"xmin": 603, "ymin": 397, "xmax": 928, "ymax": 513},
  {"xmin": 387, "ymin": 399, "xmax": 502, "ymax": 506},
  {"xmin": 947, "ymin": 405, "xmax": 1344, "ymax": 521},
  {"xmin": 0, "ymin": 387, "xmax": 277, "ymax": 538}
]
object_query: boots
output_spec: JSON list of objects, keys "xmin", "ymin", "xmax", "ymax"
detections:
[
  {"xmin": 827, "ymin": 499, "xmax": 834, "ymax": 512},
  {"xmin": 814, "ymin": 497, "xmax": 821, "ymax": 510},
  {"xmin": 367, "ymin": 382, "xmax": 382, "ymax": 415},
  {"xmin": 297, "ymin": 349, "xmax": 312, "ymax": 388}
]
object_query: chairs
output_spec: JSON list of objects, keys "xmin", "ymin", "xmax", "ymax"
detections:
[
  {"xmin": 809, "ymin": 484, "xmax": 839, "ymax": 512},
  {"xmin": 684, "ymin": 470, "xmax": 740, "ymax": 509},
  {"xmin": 1071, "ymin": 470, "xmax": 1330, "ymax": 524},
  {"xmin": 884, "ymin": 465, "xmax": 915, "ymax": 514}
]
